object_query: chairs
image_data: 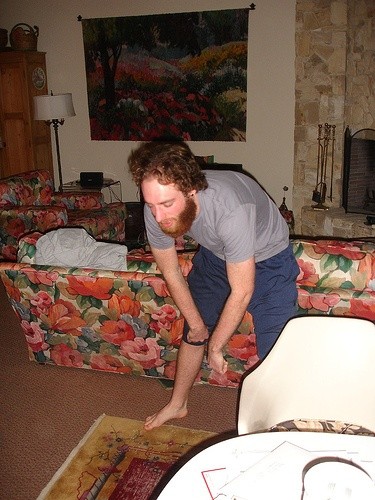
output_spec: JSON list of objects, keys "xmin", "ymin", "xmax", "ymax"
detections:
[{"xmin": 238, "ymin": 316, "xmax": 375, "ymax": 436}]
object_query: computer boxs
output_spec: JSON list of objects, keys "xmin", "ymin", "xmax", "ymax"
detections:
[{"xmin": 124, "ymin": 203, "xmax": 146, "ymax": 252}]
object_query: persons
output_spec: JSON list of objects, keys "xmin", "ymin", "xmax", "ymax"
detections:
[{"xmin": 127, "ymin": 140, "xmax": 300, "ymax": 431}]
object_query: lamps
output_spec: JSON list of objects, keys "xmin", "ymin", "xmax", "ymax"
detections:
[{"xmin": 33, "ymin": 90, "xmax": 76, "ymax": 192}]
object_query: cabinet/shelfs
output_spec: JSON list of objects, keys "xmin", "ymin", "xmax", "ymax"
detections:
[{"xmin": 0, "ymin": 50, "xmax": 55, "ymax": 192}]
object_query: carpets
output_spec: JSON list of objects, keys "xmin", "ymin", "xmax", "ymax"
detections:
[{"xmin": 36, "ymin": 412, "xmax": 238, "ymax": 500}]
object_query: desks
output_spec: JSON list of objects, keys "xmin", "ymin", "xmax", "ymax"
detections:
[
  {"xmin": 59, "ymin": 180, "xmax": 122, "ymax": 205},
  {"xmin": 156, "ymin": 432, "xmax": 375, "ymax": 500}
]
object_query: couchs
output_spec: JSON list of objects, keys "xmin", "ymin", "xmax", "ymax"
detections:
[
  {"xmin": 0, "ymin": 232, "xmax": 375, "ymax": 388},
  {"xmin": 0, "ymin": 169, "xmax": 125, "ymax": 261}
]
